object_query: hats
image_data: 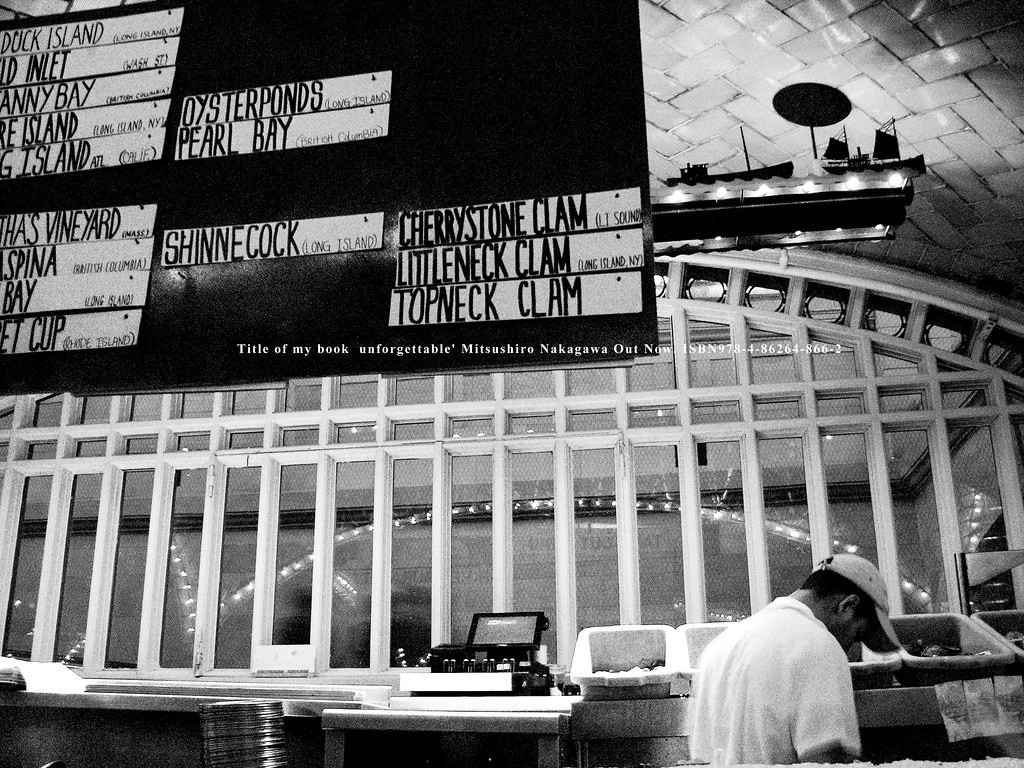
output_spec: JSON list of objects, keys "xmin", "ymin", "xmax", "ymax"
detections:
[{"xmin": 811, "ymin": 553, "xmax": 901, "ymax": 653}]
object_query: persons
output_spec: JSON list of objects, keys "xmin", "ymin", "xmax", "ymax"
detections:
[{"xmin": 687, "ymin": 555, "xmax": 902, "ymax": 765}]
